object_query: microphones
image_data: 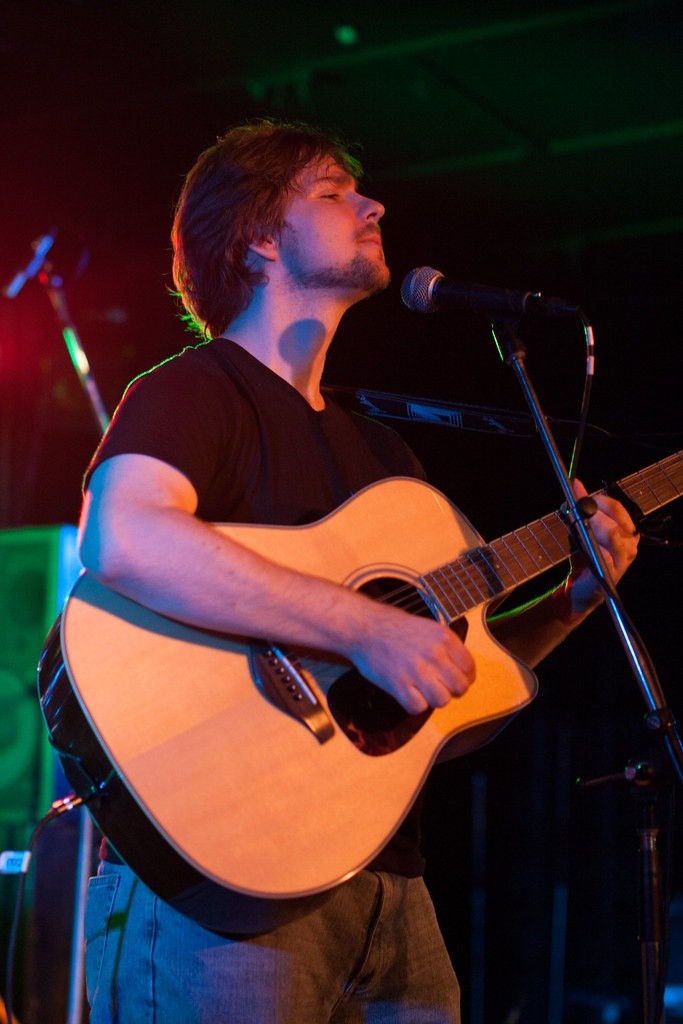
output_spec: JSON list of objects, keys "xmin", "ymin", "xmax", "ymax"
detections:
[
  {"xmin": 402, "ymin": 268, "xmax": 580, "ymax": 318},
  {"xmin": 6, "ymin": 234, "xmax": 56, "ymax": 299}
]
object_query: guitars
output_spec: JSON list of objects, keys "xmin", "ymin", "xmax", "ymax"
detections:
[{"xmin": 36, "ymin": 450, "xmax": 682, "ymax": 935}]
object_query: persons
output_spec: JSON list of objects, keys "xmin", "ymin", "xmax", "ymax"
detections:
[{"xmin": 76, "ymin": 118, "xmax": 642, "ymax": 1024}]
